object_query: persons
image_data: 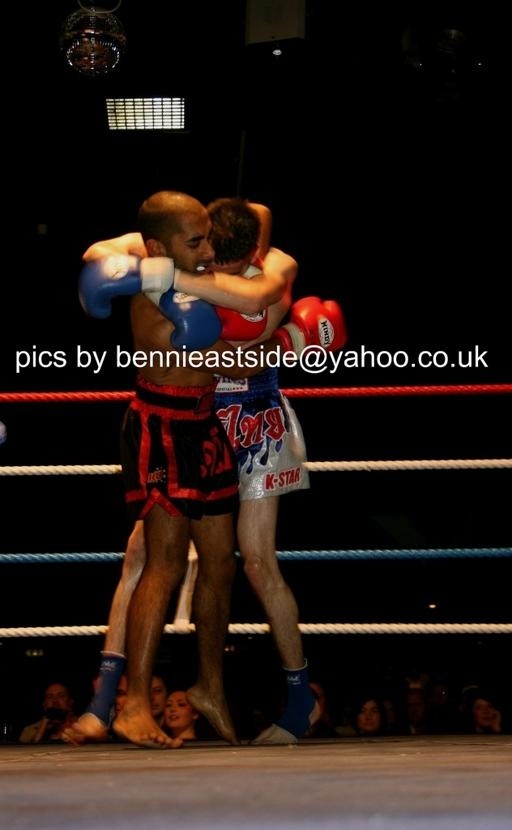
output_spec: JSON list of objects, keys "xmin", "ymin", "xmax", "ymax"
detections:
[
  {"xmin": 67, "ymin": 190, "xmax": 325, "ymax": 752},
  {"xmin": 103, "ymin": 190, "xmax": 349, "ymax": 747},
  {"xmin": 18, "ymin": 667, "xmax": 511, "ymax": 745}
]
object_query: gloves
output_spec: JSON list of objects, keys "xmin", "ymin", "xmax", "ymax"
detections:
[
  {"xmin": 142, "ymin": 289, "xmax": 222, "ymax": 351},
  {"xmin": 216, "ymin": 258, "xmax": 267, "ymax": 341},
  {"xmin": 79, "ymin": 254, "xmax": 174, "ymax": 317},
  {"xmin": 275, "ymin": 297, "xmax": 348, "ymax": 362}
]
